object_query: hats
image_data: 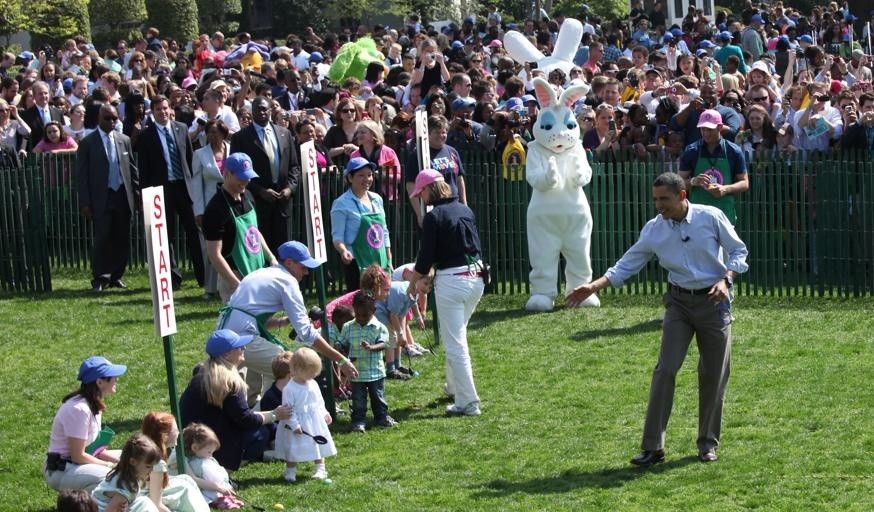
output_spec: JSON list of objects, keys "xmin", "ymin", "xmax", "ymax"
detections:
[
  {"xmin": 748, "ymin": 61, "xmax": 771, "ymax": 81},
  {"xmin": 797, "ymin": 35, "xmax": 812, "ymax": 43},
  {"xmin": 663, "ymin": 29, "xmax": 685, "ymax": 42},
  {"xmin": 77, "ymin": 356, "xmax": 126, "ymax": 385},
  {"xmin": 206, "ymin": 329, "xmax": 253, "ymax": 358},
  {"xmin": 697, "ymin": 110, "xmax": 722, "ymax": 129},
  {"xmin": 306, "ymin": 52, "xmax": 324, "ymax": 61},
  {"xmin": 506, "ymin": 94, "xmax": 536, "ymax": 110},
  {"xmin": 277, "ymin": 241, "xmax": 325, "ymax": 268},
  {"xmin": 226, "ymin": 153, "xmax": 260, "ymax": 181},
  {"xmin": 63, "ymin": 78, "xmax": 73, "ymax": 90},
  {"xmin": 771, "ymin": 122, "xmax": 794, "ymax": 136},
  {"xmin": 18, "ymin": 51, "xmax": 33, "ymax": 60},
  {"xmin": 489, "ymin": 40, "xmax": 501, "ymax": 48},
  {"xmin": 645, "ymin": 69, "xmax": 662, "ymax": 78},
  {"xmin": 752, "ymin": 14, "xmax": 765, "ymax": 24},
  {"xmin": 346, "ymin": 157, "xmax": 376, "ymax": 174},
  {"xmin": 451, "ymin": 99, "xmax": 474, "ymax": 112},
  {"xmin": 278, "ymin": 47, "xmax": 294, "ymax": 56},
  {"xmin": 410, "ymin": 169, "xmax": 443, "ymax": 199},
  {"xmin": 182, "ymin": 77, "xmax": 227, "ymax": 90},
  {"xmin": 697, "ymin": 32, "xmax": 733, "ymax": 58},
  {"xmin": 452, "ymin": 41, "xmax": 463, "ymax": 48}
]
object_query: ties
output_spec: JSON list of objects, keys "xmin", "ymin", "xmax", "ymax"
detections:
[
  {"xmin": 261, "ymin": 128, "xmax": 278, "ymax": 181},
  {"xmin": 105, "ymin": 134, "xmax": 120, "ymax": 191},
  {"xmin": 41, "ymin": 109, "xmax": 47, "ymax": 126},
  {"xmin": 163, "ymin": 127, "xmax": 182, "ymax": 180},
  {"xmin": 293, "ymin": 95, "xmax": 298, "ymax": 110}
]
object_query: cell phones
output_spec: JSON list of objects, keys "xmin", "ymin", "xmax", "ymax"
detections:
[
  {"xmin": 311, "ymin": 62, "xmax": 316, "ymax": 73},
  {"xmin": 609, "ymin": 121, "xmax": 614, "ymax": 130},
  {"xmin": 845, "ymin": 106, "xmax": 852, "ymax": 115}
]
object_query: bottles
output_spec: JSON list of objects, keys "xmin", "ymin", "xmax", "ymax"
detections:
[{"xmin": 705, "ymin": 66, "xmax": 716, "ymax": 81}]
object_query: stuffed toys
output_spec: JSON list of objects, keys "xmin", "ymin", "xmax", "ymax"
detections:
[{"xmin": 526, "ymin": 78, "xmax": 601, "ymax": 311}]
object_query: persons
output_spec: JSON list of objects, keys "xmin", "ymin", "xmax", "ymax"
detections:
[
  {"xmin": 565, "ymin": 171, "xmax": 748, "ymax": 467},
  {"xmin": 42, "ymin": 153, "xmax": 487, "ymax": 512},
  {"xmin": 1, "ymin": 1, "xmax": 874, "ymax": 299}
]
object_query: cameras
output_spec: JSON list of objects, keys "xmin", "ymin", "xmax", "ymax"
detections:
[
  {"xmin": 699, "ymin": 96, "xmax": 714, "ymax": 109},
  {"xmin": 795, "ymin": 47, "xmax": 805, "ymax": 58},
  {"xmin": 643, "ymin": 65, "xmax": 654, "ymax": 70},
  {"xmin": 706, "ymin": 57, "xmax": 714, "ymax": 63},
  {"xmin": 426, "ymin": 53, "xmax": 437, "ymax": 60},
  {"xmin": 817, "ymin": 95, "xmax": 829, "ymax": 101},
  {"xmin": 220, "ymin": 68, "xmax": 232, "ymax": 76},
  {"xmin": 306, "ymin": 109, "xmax": 315, "ymax": 115},
  {"xmin": 518, "ymin": 107, "xmax": 529, "ymax": 117},
  {"xmin": 44, "ymin": 46, "xmax": 53, "ymax": 58},
  {"xmin": 629, "ymin": 73, "xmax": 639, "ymax": 87},
  {"xmin": 282, "ymin": 111, "xmax": 292, "ymax": 119},
  {"xmin": 743, "ymin": 129, "xmax": 751, "ymax": 137},
  {"xmin": 665, "ymin": 87, "xmax": 677, "ymax": 94},
  {"xmin": 459, "ymin": 118, "xmax": 470, "ymax": 127},
  {"xmin": 833, "ymin": 57, "xmax": 840, "ymax": 63},
  {"xmin": 528, "ymin": 62, "xmax": 537, "ymax": 68},
  {"xmin": 378, "ymin": 103, "xmax": 387, "ymax": 110}
]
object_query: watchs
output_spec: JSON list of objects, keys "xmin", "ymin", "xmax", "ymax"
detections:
[{"xmin": 723, "ymin": 274, "xmax": 734, "ymax": 289}]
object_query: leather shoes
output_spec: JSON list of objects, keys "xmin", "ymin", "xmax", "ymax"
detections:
[
  {"xmin": 699, "ymin": 449, "xmax": 716, "ymax": 461},
  {"xmin": 93, "ymin": 279, "xmax": 126, "ymax": 292},
  {"xmin": 630, "ymin": 449, "xmax": 665, "ymax": 464}
]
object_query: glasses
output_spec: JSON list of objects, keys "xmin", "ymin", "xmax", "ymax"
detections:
[
  {"xmin": 104, "ymin": 115, "xmax": 118, "ymax": 121},
  {"xmin": 752, "ymin": 95, "xmax": 769, "ymax": 101},
  {"xmin": 580, "ymin": 117, "xmax": 593, "ymax": 121},
  {"xmin": 342, "ymin": 109, "xmax": 356, "ymax": 113},
  {"xmin": 472, "ymin": 60, "xmax": 481, "ymax": 63}
]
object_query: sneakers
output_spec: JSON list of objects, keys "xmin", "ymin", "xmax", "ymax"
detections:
[
  {"xmin": 386, "ymin": 343, "xmax": 429, "ymax": 380},
  {"xmin": 377, "ymin": 415, "xmax": 397, "ymax": 427},
  {"xmin": 352, "ymin": 425, "xmax": 366, "ymax": 432},
  {"xmin": 311, "ymin": 470, "xmax": 327, "ymax": 480},
  {"xmin": 209, "ymin": 477, "xmax": 243, "ymax": 509},
  {"xmin": 444, "ymin": 382, "xmax": 480, "ymax": 415},
  {"xmin": 283, "ymin": 471, "xmax": 296, "ymax": 481}
]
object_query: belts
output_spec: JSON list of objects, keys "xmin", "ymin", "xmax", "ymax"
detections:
[
  {"xmin": 453, "ymin": 272, "xmax": 481, "ymax": 277},
  {"xmin": 671, "ymin": 285, "xmax": 713, "ymax": 295}
]
